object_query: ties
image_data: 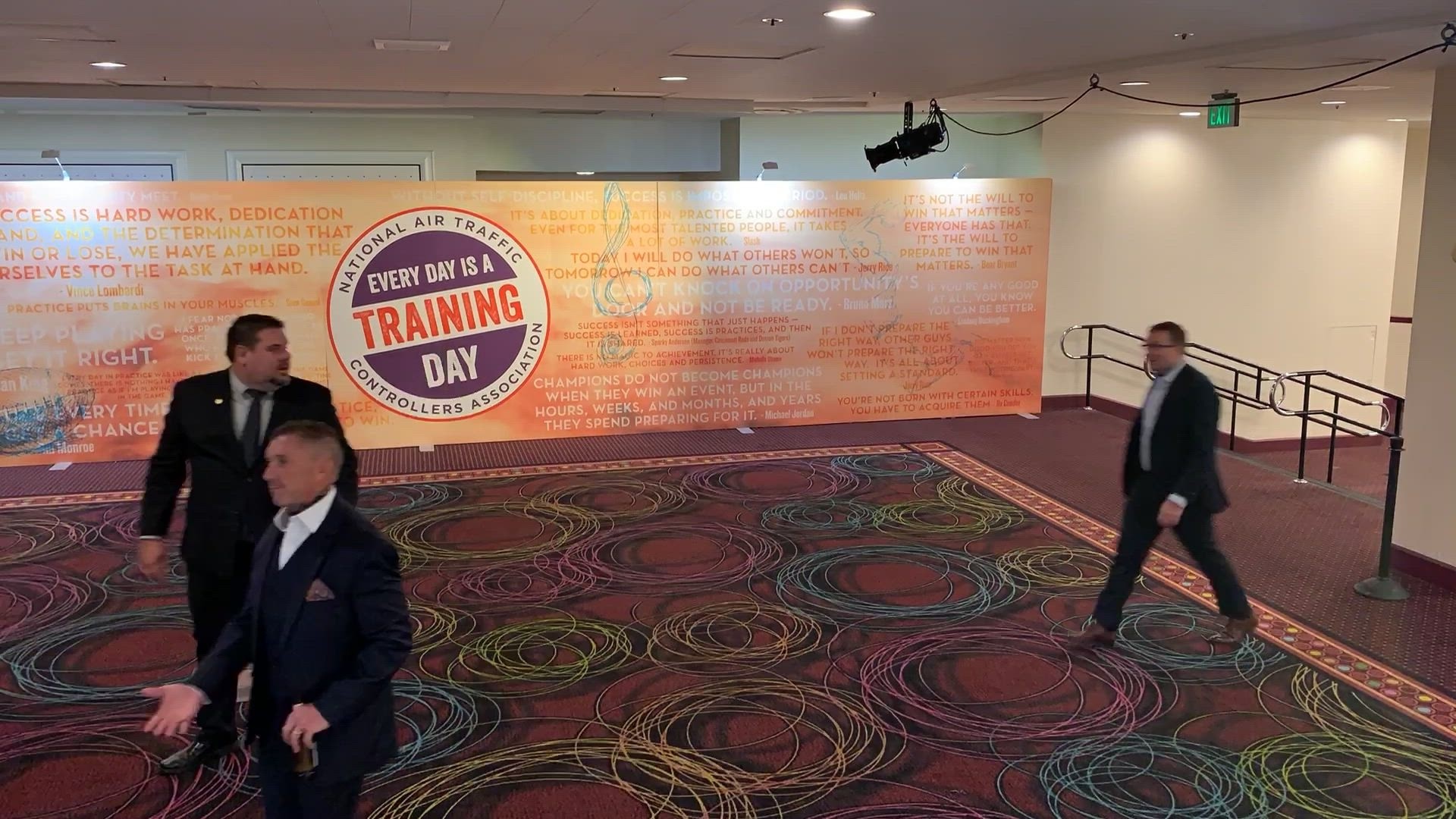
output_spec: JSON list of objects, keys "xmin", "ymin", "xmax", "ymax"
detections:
[{"xmin": 242, "ymin": 389, "xmax": 267, "ymax": 467}]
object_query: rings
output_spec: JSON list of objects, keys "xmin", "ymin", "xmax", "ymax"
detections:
[{"xmin": 291, "ymin": 731, "xmax": 301, "ymax": 735}]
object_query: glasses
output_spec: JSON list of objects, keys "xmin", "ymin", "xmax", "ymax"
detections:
[{"xmin": 1142, "ymin": 344, "xmax": 1177, "ymax": 351}]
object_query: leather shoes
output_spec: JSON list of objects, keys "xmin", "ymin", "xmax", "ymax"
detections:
[
  {"xmin": 249, "ymin": 736, "xmax": 259, "ymax": 777},
  {"xmin": 159, "ymin": 740, "xmax": 239, "ymax": 773},
  {"xmin": 1209, "ymin": 618, "xmax": 1258, "ymax": 642},
  {"xmin": 1069, "ymin": 622, "xmax": 1117, "ymax": 649}
]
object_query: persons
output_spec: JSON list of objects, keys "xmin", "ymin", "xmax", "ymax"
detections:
[
  {"xmin": 134, "ymin": 313, "xmax": 356, "ymax": 772},
  {"xmin": 136, "ymin": 423, "xmax": 410, "ymax": 819},
  {"xmin": 1071, "ymin": 320, "xmax": 1259, "ymax": 643}
]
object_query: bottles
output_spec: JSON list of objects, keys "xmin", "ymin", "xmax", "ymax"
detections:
[{"xmin": 290, "ymin": 693, "xmax": 323, "ymax": 779}]
